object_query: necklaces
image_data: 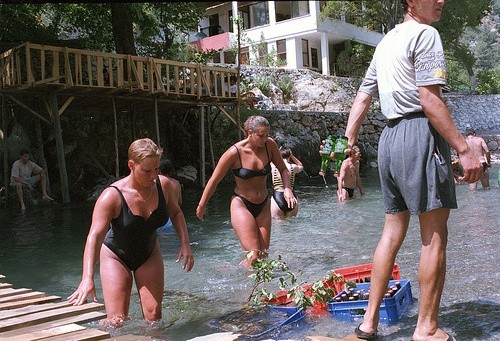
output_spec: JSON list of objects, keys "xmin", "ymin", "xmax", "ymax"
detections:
[{"xmin": 123, "ymin": 179, "xmax": 153, "ymax": 203}]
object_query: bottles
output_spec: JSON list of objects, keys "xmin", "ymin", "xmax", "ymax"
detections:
[
  {"xmin": 319, "ymin": 135, "xmax": 337, "ymax": 176},
  {"xmin": 335, "ymin": 282, "xmax": 401, "ymax": 316},
  {"xmin": 334, "ymin": 135, "xmax": 348, "ymax": 176}
]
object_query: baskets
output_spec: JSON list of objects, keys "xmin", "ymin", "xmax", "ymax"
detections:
[
  {"xmin": 326, "ymin": 279, "xmax": 412, "ymax": 325},
  {"xmin": 260, "ymin": 279, "xmax": 338, "ymax": 317},
  {"xmin": 208, "ymin": 302, "xmax": 306, "ymax": 341},
  {"xmin": 331, "ymin": 263, "xmax": 400, "ymax": 294}
]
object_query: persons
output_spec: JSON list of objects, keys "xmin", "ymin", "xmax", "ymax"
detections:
[
  {"xmin": 464, "ymin": 129, "xmax": 492, "ymax": 192},
  {"xmin": 270, "ymin": 146, "xmax": 304, "ymax": 218},
  {"xmin": 224, "ymin": 76, "xmax": 237, "ymax": 93},
  {"xmin": 337, "ymin": 146, "xmax": 365, "ymax": 201},
  {"xmin": 67, "ymin": 138, "xmax": 194, "ymax": 328},
  {"xmin": 10, "ymin": 149, "xmax": 54, "ymax": 210},
  {"xmin": 196, "ymin": 116, "xmax": 298, "ymax": 268},
  {"xmin": 319, "ymin": 0, "xmax": 483, "ymax": 341},
  {"xmin": 217, "ymin": 25, "xmax": 225, "ymax": 35},
  {"xmin": 195, "ymin": 28, "xmax": 207, "ymax": 38},
  {"xmin": 155, "ymin": 159, "xmax": 182, "ymax": 234},
  {"xmin": 102, "ymin": 68, "xmax": 118, "ymax": 86}
]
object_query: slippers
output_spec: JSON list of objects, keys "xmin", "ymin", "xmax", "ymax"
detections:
[
  {"xmin": 410, "ymin": 332, "xmax": 456, "ymax": 341},
  {"xmin": 355, "ymin": 323, "xmax": 377, "ymax": 340}
]
